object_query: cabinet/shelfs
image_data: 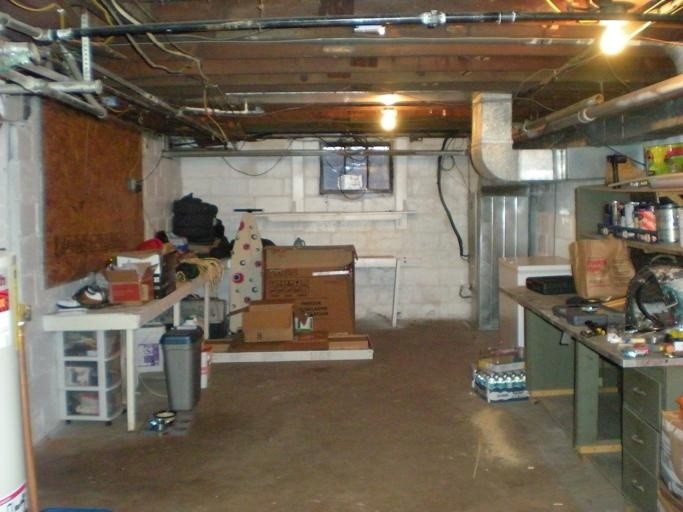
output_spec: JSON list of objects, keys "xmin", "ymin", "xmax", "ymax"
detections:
[{"xmin": 576, "ymin": 185, "xmax": 683, "ymax": 257}]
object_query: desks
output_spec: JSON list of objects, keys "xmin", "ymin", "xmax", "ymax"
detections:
[
  {"xmin": 498, "ymin": 283, "xmax": 681, "ymax": 510},
  {"xmin": 46, "ymin": 257, "xmax": 228, "ymax": 427}
]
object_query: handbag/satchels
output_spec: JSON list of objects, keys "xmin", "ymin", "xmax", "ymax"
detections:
[
  {"xmin": 172, "ymin": 216, "xmax": 213, "ymax": 238},
  {"xmin": 171, "ymin": 193, "xmax": 218, "ymax": 216}
]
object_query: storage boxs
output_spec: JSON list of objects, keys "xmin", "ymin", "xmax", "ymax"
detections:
[
  {"xmin": 54, "ymin": 329, "xmax": 126, "ymax": 425},
  {"xmin": 241, "ymin": 245, "xmax": 357, "ymax": 345},
  {"xmin": 133, "ymin": 322, "xmax": 166, "ymax": 373}
]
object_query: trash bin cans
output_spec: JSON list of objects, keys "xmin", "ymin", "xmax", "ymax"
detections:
[{"xmin": 160, "ymin": 330, "xmax": 200, "ymax": 410}]
